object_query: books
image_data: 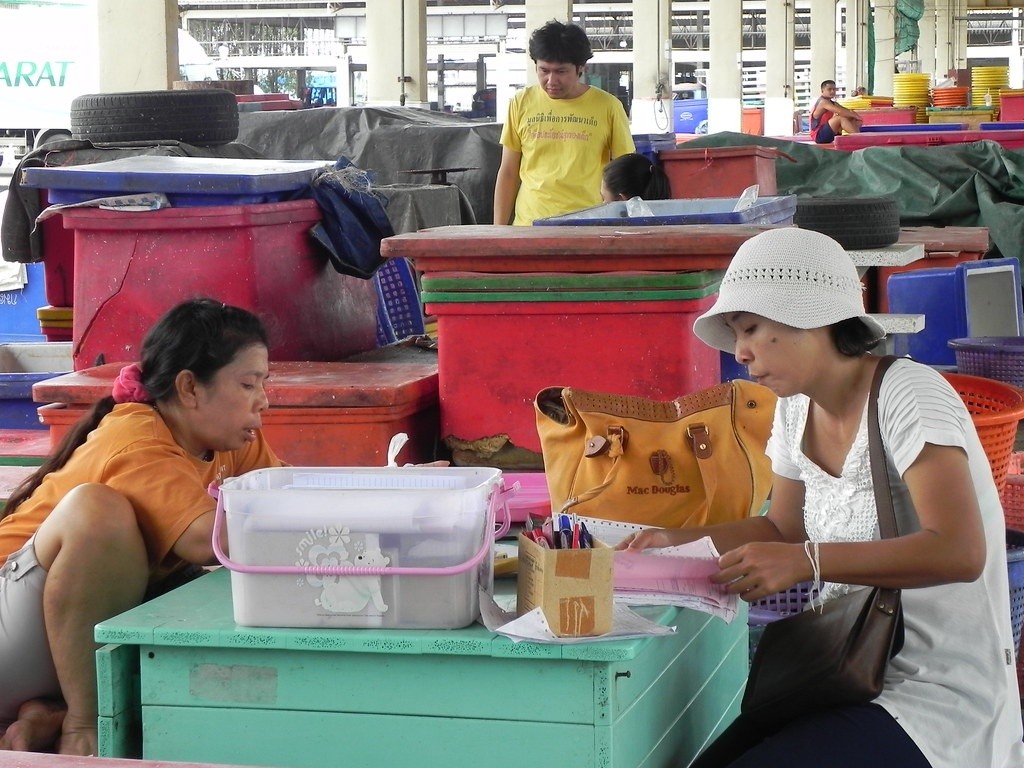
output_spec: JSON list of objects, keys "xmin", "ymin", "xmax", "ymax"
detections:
[{"xmin": 244, "ymin": 463, "xmax": 472, "ymax": 627}]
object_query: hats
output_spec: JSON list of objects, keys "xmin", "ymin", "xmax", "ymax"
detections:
[{"xmin": 693, "ymin": 229, "xmax": 886, "ymax": 356}]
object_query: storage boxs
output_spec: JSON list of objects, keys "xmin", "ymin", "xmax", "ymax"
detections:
[
  {"xmin": 0, "ymin": 64, "xmax": 1024, "ymax": 372},
  {"xmin": 208, "ymin": 466, "xmax": 520, "ymax": 631},
  {"xmin": 516, "ymin": 531, "xmax": 615, "ymax": 636},
  {"xmin": 33, "ymin": 361, "xmax": 440, "ymax": 467},
  {"xmin": 425, "ymin": 292, "xmax": 721, "ymax": 453}
]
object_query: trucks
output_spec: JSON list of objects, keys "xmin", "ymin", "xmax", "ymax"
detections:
[{"xmin": 1, "ymin": 0, "xmax": 221, "ymax": 178}]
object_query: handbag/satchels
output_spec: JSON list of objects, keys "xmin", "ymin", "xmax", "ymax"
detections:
[
  {"xmin": 742, "ymin": 585, "xmax": 906, "ymax": 732},
  {"xmin": 534, "ymin": 379, "xmax": 778, "ymax": 530}
]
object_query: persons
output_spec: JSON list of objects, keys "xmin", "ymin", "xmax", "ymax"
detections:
[
  {"xmin": 614, "ymin": 228, "xmax": 1019, "ymax": 768},
  {"xmin": 0, "ymin": 297, "xmax": 451, "ymax": 755},
  {"xmin": 599, "ymin": 153, "xmax": 669, "ymax": 203},
  {"xmin": 493, "ymin": 20, "xmax": 637, "ymax": 226},
  {"xmin": 808, "ymin": 80, "xmax": 863, "ymax": 143}
]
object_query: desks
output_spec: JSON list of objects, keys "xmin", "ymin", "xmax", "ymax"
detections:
[{"xmin": 94, "ymin": 499, "xmax": 771, "ymax": 768}]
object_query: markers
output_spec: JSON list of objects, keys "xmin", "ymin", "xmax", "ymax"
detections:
[
  {"xmin": 531, "ymin": 527, "xmax": 550, "ymax": 549},
  {"xmin": 558, "ymin": 515, "xmax": 573, "ymax": 550}
]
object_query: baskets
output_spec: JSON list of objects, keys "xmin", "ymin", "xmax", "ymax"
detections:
[
  {"xmin": 372, "ymin": 257, "xmax": 425, "ymax": 347},
  {"xmin": 748, "ymin": 336, "xmax": 1023, "ymax": 674}
]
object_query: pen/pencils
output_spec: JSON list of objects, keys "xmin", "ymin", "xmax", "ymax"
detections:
[
  {"xmin": 582, "ymin": 522, "xmax": 591, "ymax": 549},
  {"xmin": 572, "ymin": 513, "xmax": 580, "ymax": 549}
]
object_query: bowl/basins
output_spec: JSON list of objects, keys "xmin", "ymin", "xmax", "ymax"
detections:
[{"xmin": 839, "ymin": 66, "xmax": 1024, "ymax": 135}]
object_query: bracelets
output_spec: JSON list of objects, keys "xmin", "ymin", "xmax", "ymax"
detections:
[{"xmin": 804, "ymin": 540, "xmax": 823, "ymax": 614}]
